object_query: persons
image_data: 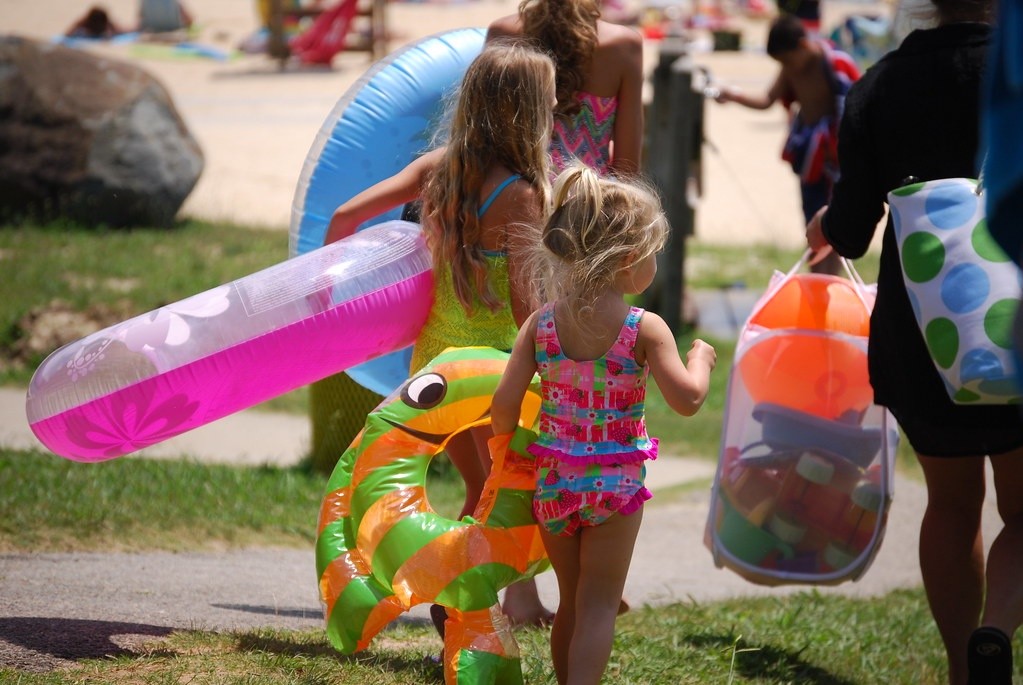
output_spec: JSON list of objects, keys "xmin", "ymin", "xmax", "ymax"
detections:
[
  {"xmin": 68, "ymin": 6, "xmax": 122, "ymax": 39},
  {"xmin": 305, "ymin": 40, "xmax": 566, "ymax": 643},
  {"xmin": 807, "ymin": 0, "xmax": 1023, "ymax": 685},
  {"xmin": 133, "ymin": 0, "xmax": 192, "ymax": 34},
  {"xmin": 488, "ymin": 165, "xmax": 720, "ymax": 685},
  {"xmin": 483, "ymin": 1, "xmax": 647, "ymax": 204},
  {"xmin": 711, "ymin": 1, "xmax": 860, "ymax": 277}
]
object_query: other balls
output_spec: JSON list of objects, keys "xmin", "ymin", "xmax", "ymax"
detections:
[{"xmin": 740, "ymin": 273, "xmax": 875, "ymax": 422}]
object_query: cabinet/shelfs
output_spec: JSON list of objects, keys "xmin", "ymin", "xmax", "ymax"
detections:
[{"xmin": 271, "ymin": 0, "xmax": 386, "ymax": 70}]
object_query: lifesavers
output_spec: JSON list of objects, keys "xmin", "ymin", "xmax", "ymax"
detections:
[
  {"xmin": 310, "ymin": 342, "xmax": 547, "ymax": 681},
  {"xmin": 22, "ymin": 221, "xmax": 440, "ymax": 464},
  {"xmin": 288, "ymin": 24, "xmax": 493, "ymax": 403}
]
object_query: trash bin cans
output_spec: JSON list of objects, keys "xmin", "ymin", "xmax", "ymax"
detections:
[{"xmin": 712, "ymin": 31, "xmax": 743, "ymax": 51}]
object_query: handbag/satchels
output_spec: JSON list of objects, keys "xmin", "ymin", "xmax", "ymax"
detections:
[{"xmin": 888, "ymin": 178, "xmax": 1023, "ymax": 405}]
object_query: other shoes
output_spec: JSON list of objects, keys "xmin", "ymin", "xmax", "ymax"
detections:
[{"xmin": 969, "ymin": 626, "xmax": 1013, "ymax": 685}]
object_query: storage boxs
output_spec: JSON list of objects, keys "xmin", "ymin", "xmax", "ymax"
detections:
[{"xmin": 715, "ymin": 33, "xmax": 739, "ymax": 49}]
